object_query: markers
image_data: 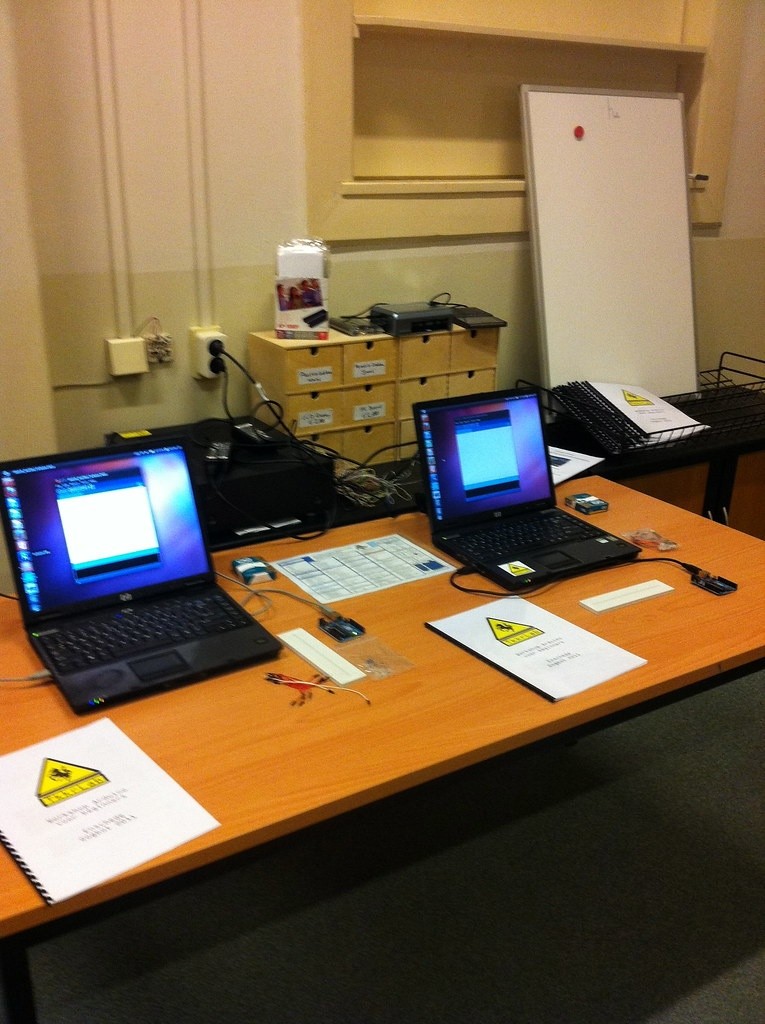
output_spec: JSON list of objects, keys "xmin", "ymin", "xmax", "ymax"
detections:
[{"xmin": 689, "ymin": 173, "xmax": 709, "ymax": 181}]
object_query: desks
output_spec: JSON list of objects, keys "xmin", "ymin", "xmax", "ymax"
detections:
[{"xmin": 0, "ymin": 472, "xmax": 765, "ymax": 1024}]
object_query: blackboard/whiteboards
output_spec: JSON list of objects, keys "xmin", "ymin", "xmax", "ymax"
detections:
[{"xmin": 519, "ymin": 84, "xmax": 702, "ymax": 424}]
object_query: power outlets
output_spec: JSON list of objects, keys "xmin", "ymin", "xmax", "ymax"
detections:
[{"xmin": 188, "ymin": 325, "xmax": 228, "ymax": 381}]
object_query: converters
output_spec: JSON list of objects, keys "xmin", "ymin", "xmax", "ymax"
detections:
[{"xmin": 203, "ymin": 437, "xmax": 235, "ymax": 475}]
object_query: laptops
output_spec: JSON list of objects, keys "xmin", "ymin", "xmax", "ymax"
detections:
[
  {"xmin": 412, "ymin": 387, "xmax": 640, "ymax": 592},
  {"xmin": 0, "ymin": 436, "xmax": 283, "ymax": 715}
]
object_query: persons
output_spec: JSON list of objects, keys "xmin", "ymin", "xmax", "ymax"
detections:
[{"xmin": 278, "ymin": 278, "xmax": 322, "ymax": 310}]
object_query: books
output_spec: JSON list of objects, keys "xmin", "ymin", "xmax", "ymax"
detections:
[
  {"xmin": 1, "ymin": 714, "xmax": 223, "ymax": 908},
  {"xmin": 552, "ymin": 379, "xmax": 712, "ymax": 461},
  {"xmin": 423, "ymin": 593, "xmax": 649, "ymax": 705}
]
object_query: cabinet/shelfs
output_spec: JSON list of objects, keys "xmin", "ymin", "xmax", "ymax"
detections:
[{"xmin": 248, "ymin": 322, "xmax": 500, "ymax": 468}]
